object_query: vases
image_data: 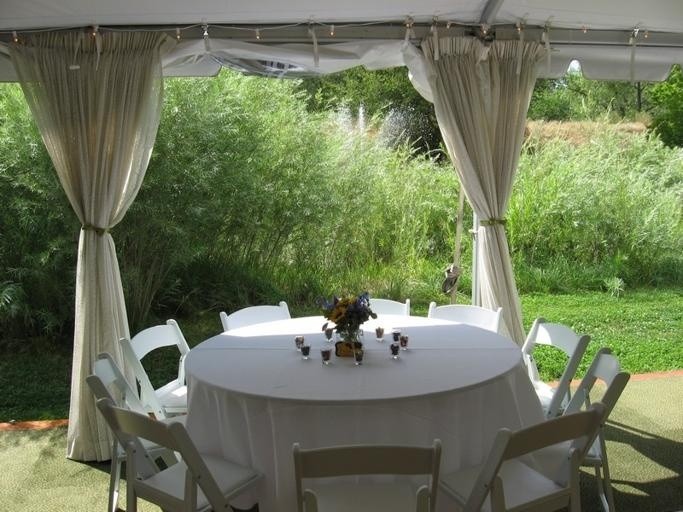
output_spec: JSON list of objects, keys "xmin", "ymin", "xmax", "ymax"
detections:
[{"xmin": 335, "ymin": 342, "xmax": 362, "ymax": 357}]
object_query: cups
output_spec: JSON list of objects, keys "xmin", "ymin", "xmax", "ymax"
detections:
[
  {"xmin": 295, "ymin": 336, "xmax": 304, "ymax": 352},
  {"xmin": 375, "ymin": 327, "xmax": 385, "ymax": 340},
  {"xmin": 390, "ymin": 343, "xmax": 401, "ymax": 359},
  {"xmin": 300, "ymin": 344, "xmax": 311, "ymax": 360},
  {"xmin": 399, "ymin": 336, "xmax": 409, "ymax": 350},
  {"xmin": 354, "ymin": 349, "xmax": 364, "ymax": 366},
  {"xmin": 320, "ymin": 349, "xmax": 332, "ymax": 365},
  {"xmin": 392, "ymin": 330, "xmax": 402, "ymax": 344}
]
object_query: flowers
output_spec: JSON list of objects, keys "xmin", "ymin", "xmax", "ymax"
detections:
[{"xmin": 323, "ymin": 292, "xmax": 377, "ymax": 342}]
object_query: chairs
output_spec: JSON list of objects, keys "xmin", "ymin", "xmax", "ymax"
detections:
[
  {"xmin": 428, "ymin": 302, "xmax": 503, "ymax": 333},
  {"xmin": 562, "ymin": 348, "xmax": 629, "ymax": 512},
  {"xmin": 293, "ymin": 438, "xmax": 441, "ymax": 512},
  {"xmin": 339, "ymin": 297, "xmax": 411, "ymax": 315},
  {"xmin": 441, "ymin": 400, "xmax": 604, "ymax": 512},
  {"xmin": 95, "ymin": 398, "xmax": 264, "ymax": 512},
  {"xmin": 220, "ymin": 301, "xmax": 291, "ymax": 331},
  {"xmin": 87, "ymin": 352, "xmax": 180, "ymax": 512},
  {"xmin": 522, "ymin": 319, "xmax": 589, "ymax": 419},
  {"xmin": 119, "ymin": 319, "xmax": 191, "ymax": 420}
]
